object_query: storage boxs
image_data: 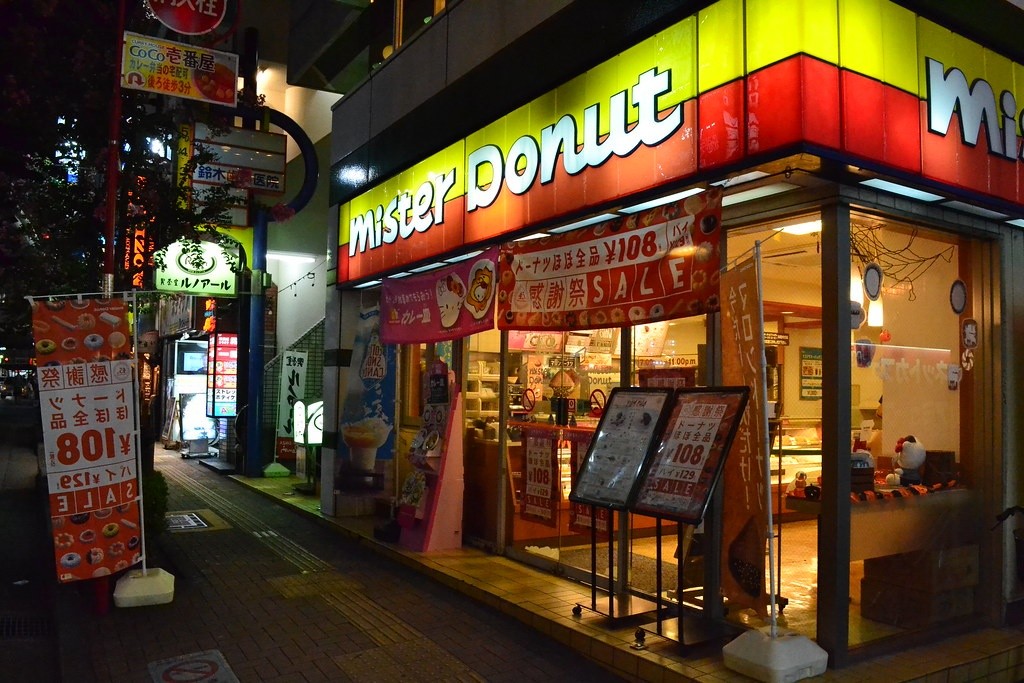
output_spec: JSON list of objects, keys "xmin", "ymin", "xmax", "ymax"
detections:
[{"xmin": 860, "ymin": 543, "xmax": 979, "ymax": 629}]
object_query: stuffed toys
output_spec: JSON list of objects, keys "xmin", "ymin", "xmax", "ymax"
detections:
[{"xmin": 886, "ymin": 435, "xmax": 926, "ymax": 487}]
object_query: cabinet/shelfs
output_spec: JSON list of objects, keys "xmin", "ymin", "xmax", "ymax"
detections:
[{"xmin": 419, "ymin": 343, "xmax": 522, "ymax": 430}]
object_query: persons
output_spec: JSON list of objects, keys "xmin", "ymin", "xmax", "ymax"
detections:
[{"xmin": 4, "ymin": 372, "xmax": 39, "ymax": 404}]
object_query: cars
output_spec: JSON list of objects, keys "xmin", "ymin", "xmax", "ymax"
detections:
[{"xmin": 0, "ymin": 375, "xmax": 32, "ymax": 399}]
